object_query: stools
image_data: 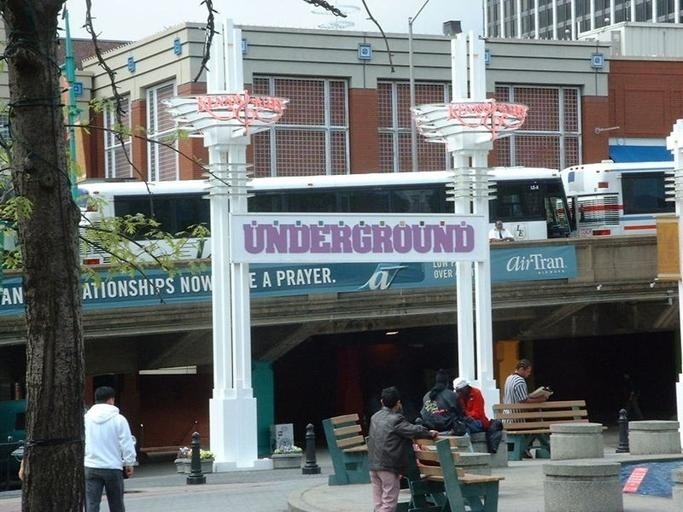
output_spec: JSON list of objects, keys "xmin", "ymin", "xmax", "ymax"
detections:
[
  {"xmin": 672, "ymin": 469, "xmax": 683, "ymax": 512},
  {"xmin": 437, "ymin": 431, "xmax": 508, "ymax": 499},
  {"xmin": 542, "ymin": 461, "xmax": 623, "ymax": 511},
  {"xmin": 628, "ymin": 420, "xmax": 683, "ymax": 455},
  {"xmin": 549, "ymin": 422, "xmax": 603, "ymax": 461}
]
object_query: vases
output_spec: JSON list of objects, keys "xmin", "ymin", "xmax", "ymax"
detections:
[
  {"xmin": 184, "ymin": 460, "xmax": 213, "ymax": 473},
  {"xmin": 174, "ymin": 458, "xmax": 185, "ymax": 473},
  {"xmin": 271, "ymin": 453, "xmax": 303, "ymax": 469}
]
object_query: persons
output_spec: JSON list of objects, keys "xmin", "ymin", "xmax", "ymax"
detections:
[
  {"xmin": 365, "ymin": 386, "xmax": 440, "ymax": 512},
  {"xmin": 501, "ymin": 358, "xmax": 549, "ymax": 459},
  {"xmin": 83, "ymin": 386, "xmax": 139, "ymax": 511},
  {"xmin": 419, "ymin": 369, "xmax": 466, "ymax": 433},
  {"xmin": 622, "ymin": 370, "xmax": 645, "ymax": 420},
  {"xmin": 452, "ymin": 376, "xmax": 490, "ymax": 434}
]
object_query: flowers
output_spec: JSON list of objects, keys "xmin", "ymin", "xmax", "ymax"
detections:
[
  {"xmin": 188, "ymin": 449, "xmax": 215, "ymax": 461},
  {"xmin": 273, "ymin": 445, "xmax": 303, "ymax": 454},
  {"xmin": 176, "ymin": 446, "xmax": 189, "ymax": 458}
]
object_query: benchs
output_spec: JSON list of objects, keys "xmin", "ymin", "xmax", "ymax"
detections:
[
  {"xmin": 322, "ymin": 413, "xmax": 372, "ymax": 485},
  {"xmin": 492, "ymin": 400, "xmax": 609, "ymax": 461},
  {"xmin": 397, "ymin": 437, "xmax": 505, "ymax": 512}
]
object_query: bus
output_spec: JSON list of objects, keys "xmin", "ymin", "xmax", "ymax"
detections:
[
  {"xmin": 75, "ymin": 162, "xmax": 580, "ymax": 264},
  {"xmin": 559, "ymin": 158, "xmax": 683, "ymax": 240}
]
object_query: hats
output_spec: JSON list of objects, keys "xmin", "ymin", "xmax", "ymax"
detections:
[{"xmin": 452, "ymin": 377, "xmax": 470, "ymax": 391}]
